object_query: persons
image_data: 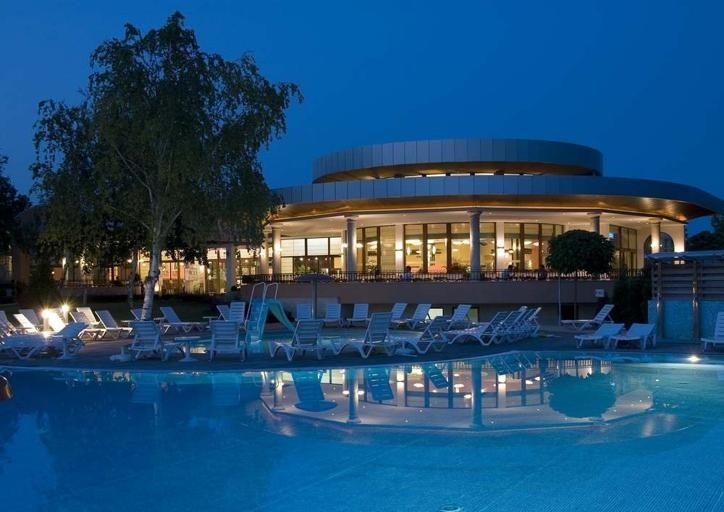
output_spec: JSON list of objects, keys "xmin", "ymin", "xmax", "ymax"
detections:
[
  {"xmin": 403, "ymin": 265, "xmax": 414, "ymax": 282},
  {"xmin": 503, "ymin": 264, "xmax": 515, "ymax": 282},
  {"xmin": 537, "ymin": 262, "xmax": 548, "ymax": 280}
]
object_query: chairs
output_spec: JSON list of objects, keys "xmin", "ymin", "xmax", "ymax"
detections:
[
  {"xmin": 128, "ymin": 321, "xmax": 179, "ymax": 362},
  {"xmin": 160, "ymin": 305, "xmax": 209, "ymax": 334},
  {"xmin": 346, "ymin": 303, "xmax": 370, "ymax": 325},
  {"xmin": 331, "ymin": 312, "xmax": 398, "ymax": 360},
  {"xmin": 267, "ymin": 318, "xmax": 330, "ymax": 361},
  {"xmin": 204, "ymin": 318, "xmax": 248, "ymax": 362},
  {"xmin": 323, "ymin": 303, "xmax": 342, "ymax": 328},
  {"xmin": 230, "ymin": 300, "xmax": 246, "ymax": 321},
  {"xmin": 449, "ymin": 304, "xmax": 542, "ymax": 347},
  {"xmin": 217, "ymin": 304, "xmax": 232, "ymax": 321},
  {"xmin": 562, "ymin": 305, "xmax": 658, "ymax": 350},
  {"xmin": 392, "ymin": 303, "xmax": 434, "ymax": 328},
  {"xmin": 366, "ymin": 301, "xmax": 409, "ymax": 327},
  {"xmin": 290, "ymin": 353, "xmax": 545, "ymax": 411},
  {"xmin": 395, "ymin": 313, "xmax": 451, "ymax": 354},
  {"xmin": 1, "ymin": 305, "xmax": 132, "ymax": 362},
  {"xmin": 296, "ymin": 302, "xmax": 315, "ymax": 320},
  {"xmin": 701, "ymin": 311, "xmax": 724, "ymax": 351},
  {"xmin": 129, "ymin": 307, "xmax": 172, "ymax": 337}
]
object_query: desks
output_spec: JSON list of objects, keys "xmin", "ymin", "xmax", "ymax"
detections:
[{"xmin": 174, "ymin": 336, "xmax": 203, "ymax": 362}]
